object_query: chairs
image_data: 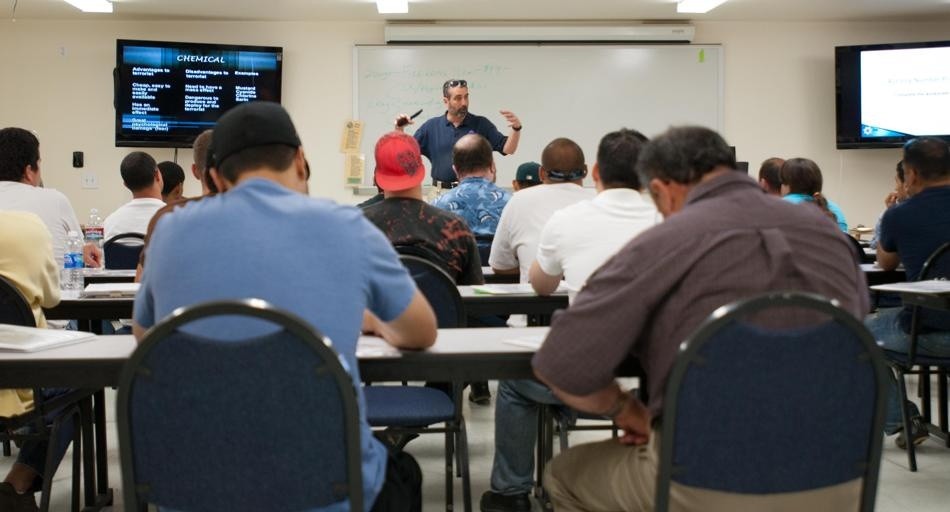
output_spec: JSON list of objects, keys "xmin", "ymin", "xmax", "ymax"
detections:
[{"xmin": 0, "ymin": 232, "xmax": 950, "ymax": 512}]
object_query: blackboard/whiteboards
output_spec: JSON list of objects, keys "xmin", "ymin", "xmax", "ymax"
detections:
[{"xmin": 351, "ymin": 42, "xmax": 726, "ymax": 197}]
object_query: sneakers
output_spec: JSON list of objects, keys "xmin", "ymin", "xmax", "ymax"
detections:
[
  {"xmin": 482, "ymin": 489, "xmax": 527, "ymax": 511},
  {"xmin": 384, "ymin": 429, "xmax": 404, "ymax": 450},
  {"xmin": 469, "ymin": 391, "xmax": 490, "ymax": 402},
  {"xmin": 896, "ymin": 417, "xmax": 926, "ymax": 449},
  {"xmin": 0, "ymin": 481, "xmax": 39, "ymax": 511}
]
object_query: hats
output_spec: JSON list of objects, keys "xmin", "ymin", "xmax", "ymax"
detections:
[
  {"xmin": 516, "ymin": 162, "xmax": 539, "ymax": 181},
  {"xmin": 212, "ymin": 102, "xmax": 309, "ymax": 178},
  {"xmin": 374, "ymin": 134, "xmax": 426, "ymax": 190}
]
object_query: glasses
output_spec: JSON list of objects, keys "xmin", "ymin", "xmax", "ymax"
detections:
[
  {"xmin": 446, "ymin": 80, "xmax": 467, "ymax": 88},
  {"xmin": 544, "ymin": 168, "xmax": 583, "ymax": 181}
]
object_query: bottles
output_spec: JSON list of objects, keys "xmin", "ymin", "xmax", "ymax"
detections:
[{"xmin": 62, "ymin": 208, "xmax": 105, "ymax": 299}]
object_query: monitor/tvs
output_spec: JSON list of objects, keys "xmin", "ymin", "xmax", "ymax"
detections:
[
  {"xmin": 114, "ymin": 38, "xmax": 283, "ymax": 149},
  {"xmin": 833, "ymin": 40, "xmax": 949, "ymax": 149}
]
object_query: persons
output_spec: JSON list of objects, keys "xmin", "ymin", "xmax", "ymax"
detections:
[
  {"xmin": 479, "ymin": 128, "xmax": 671, "ymax": 511},
  {"xmin": 394, "ymin": 78, "xmax": 522, "ymax": 206},
  {"xmin": 488, "ymin": 137, "xmax": 597, "ymax": 326},
  {"xmin": 532, "ymin": 123, "xmax": 872, "ymax": 510},
  {"xmin": 2, "ymin": 126, "xmax": 116, "ymax": 336},
  {"xmin": 100, "ymin": 151, "xmax": 169, "ymax": 247},
  {"xmin": 759, "ymin": 157, "xmax": 786, "ymax": 199},
  {"xmin": 513, "ymin": 161, "xmax": 543, "ymax": 195},
  {"xmin": 870, "ymin": 159, "xmax": 911, "ymax": 248},
  {"xmin": 356, "ymin": 166, "xmax": 385, "ymax": 206},
  {"xmin": 135, "ymin": 129, "xmax": 215, "ymax": 283},
  {"xmin": 2, "ymin": 207, "xmax": 82, "ymax": 512},
  {"xmin": 360, "ymin": 131, "xmax": 487, "ymax": 444},
  {"xmin": 435, "ymin": 134, "xmax": 516, "ymax": 400},
  {"xmin": 135, "ymin": 101, "xmax": 424, "ymax": 511},
  {"xmin": 780, "ymin": 157, "xmax": 848, "ymax": 234},
  {"xmin": 864, "ymin": 135, "xmax": 950, "ymax": 449},
  {"xmin": 157, "ymin": 161, "xmax": 189, "ymax": 206}
]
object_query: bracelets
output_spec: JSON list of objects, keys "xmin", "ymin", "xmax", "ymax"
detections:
[{"xmin": 512, "ymin": 126, "xmax": 521, "ymax": 131}]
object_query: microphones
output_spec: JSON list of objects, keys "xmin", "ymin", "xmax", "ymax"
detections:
[{"xmin": 397, "ymin": 110, "xmax": 424, "ymax": 127}]
